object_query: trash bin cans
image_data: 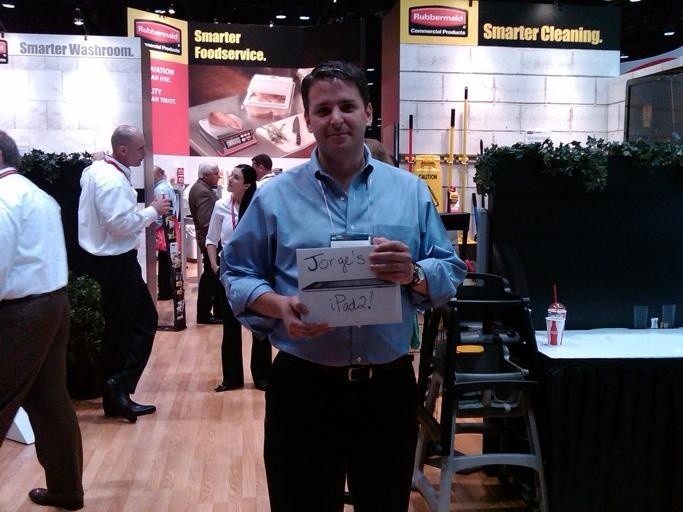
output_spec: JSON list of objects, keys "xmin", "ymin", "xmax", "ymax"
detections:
[{"xmin": 184, "ymin": 215, "xmax": 204, "ymax": 263}]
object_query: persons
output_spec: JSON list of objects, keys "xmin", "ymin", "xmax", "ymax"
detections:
[
  {"xmin": 251, "ymin": 153, "xmax": 277, "ymax": 190},
  {"xmin": 77, "ymin": 124, "xmax": 171, "ymax": 423},
  {"xmin": 204, "ymin": 163, "xmax": 272, "ymax": 392},
  {"xmin": 188, "ymin": 160, "xmax": 225, "ymax": 325},
  {"xmin": 154, "ymin": 166, "xmax": 176, "ymax": 301},
  {"xmin": 364, "ymin": 138, "xmax": 394, "ymax": 165},
  {"xmin": 388, "ymin": 154, "xmax": 400, "ymax": 168},
  {"xmin": 219, "ymin": 62, "xmax": 467, "ymax": 512},
  {"xmin": 0, "ymin": 131, "xmax": 84, "ymax": 511},
  {"xmin": 410, "ymin": 313, "xmax": 421, "ymax": 354}
]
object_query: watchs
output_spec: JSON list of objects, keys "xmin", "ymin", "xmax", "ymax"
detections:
[{"xmin": 411, "ymin": 263, "xmax": 425, "ymax": 288}]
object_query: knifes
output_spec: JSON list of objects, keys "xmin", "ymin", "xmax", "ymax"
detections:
[{"xmin": 292, "ymin": 115, "xmax": 302, "ymax": 147}]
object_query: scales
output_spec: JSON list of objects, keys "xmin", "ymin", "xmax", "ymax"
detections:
[{"xmin": 198, "ymin": 114, "xmax": 257, "ymax": 156}]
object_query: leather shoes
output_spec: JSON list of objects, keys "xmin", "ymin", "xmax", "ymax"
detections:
[
  {"xmin": 196, "ymin": 313, "xmax": 223, "ymax": 325},
  {"xmin": 29, "ymin": 487, "xmax": 84, "ymax": 510},
  {"xmin": 102, "ymin": 381, "xmax": 156, "ymax": 424}
]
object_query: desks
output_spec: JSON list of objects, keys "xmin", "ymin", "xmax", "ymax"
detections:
[{"xmin": 482, "ymin": 326, "xmax": 683, "ymax": 512}]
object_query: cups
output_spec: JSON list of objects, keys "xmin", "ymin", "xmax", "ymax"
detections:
[{"xmin": 544, "ymin": 309, "xmax": 567, "ymax": 346}]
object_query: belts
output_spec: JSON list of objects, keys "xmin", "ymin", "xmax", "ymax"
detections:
[
  {"xmin": 278, "ymin": 351, "xmax": 412, "ymax": 382},
  {"xmin": 0, "ymin": 285, "xmax": 66, "ymax": 305}
]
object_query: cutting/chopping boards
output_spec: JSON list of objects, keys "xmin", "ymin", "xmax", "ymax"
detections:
[{"xmin": 254, "ymin": 114, "xmax": 317, "ymax": 155}]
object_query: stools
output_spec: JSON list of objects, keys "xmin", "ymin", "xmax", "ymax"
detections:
[{"xmin": 410, "ymin": 272, "xmax": 551, "ymax": 512}]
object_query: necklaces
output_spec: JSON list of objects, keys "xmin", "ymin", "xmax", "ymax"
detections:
[
  {"xmin": 104, "ymin": 157, "xmax": 131, "ymax": 181},
  {"xmin": 0, "ymin": 170, "xmax": 18, "ymax": 178},
  {"xmin": 232, "ymin": 199, "xmax": 238, "ymax": 231},
  {"xmin": 318, "ymin": 176, "xmax": 372, "ymax": 247}
]
